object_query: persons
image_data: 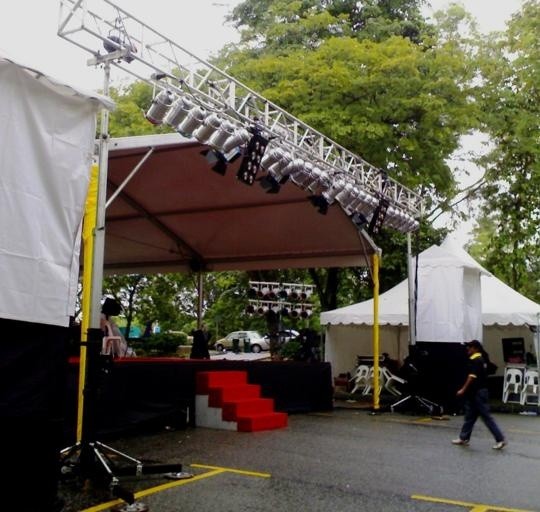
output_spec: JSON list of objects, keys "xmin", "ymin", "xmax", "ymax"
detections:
[
  {"xmin": 190, "ymin": 323, "xmax": 212, "ymax": 360},
  {"xmin": 451, "ymin": 340, "xmax": 508, "ymax": 451}
]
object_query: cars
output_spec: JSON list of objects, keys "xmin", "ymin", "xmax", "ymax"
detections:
[{"xmin": 207, "ymin": 327, "xmax": 306, "ymax": 353}]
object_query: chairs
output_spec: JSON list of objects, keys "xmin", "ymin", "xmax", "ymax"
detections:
[
  {"xmin": 502, "ymin": 369, "xmax": 523, "ymax": 404},
  {"xmin": 520, "ymin": 371, "xmax": 540, "ymax": 407},
  {"xmin": 365, "ymin": 366, "xmax": 385, "ymax": 395},
  {"xmin": 348, "ymin": 365, "xmax": 370, "ymax": 395},
  {"xmin": 382, "ymin": 366, "xmax": 408, "ymax": 396}
]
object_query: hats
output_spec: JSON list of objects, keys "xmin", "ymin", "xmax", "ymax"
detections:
[{"xmin": 465, "ymin": 341, "xmax": 480, "ymax": 348}]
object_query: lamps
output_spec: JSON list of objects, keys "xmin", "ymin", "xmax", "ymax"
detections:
[{"xmin": 246, "ymin": 283, "xmax": 313, "ymax": 320}]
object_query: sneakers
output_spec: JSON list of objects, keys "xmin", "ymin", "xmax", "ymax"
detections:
[
  {"xmin": 452, "ymin": 439, "xmax": 467, "ymax": 444},
  {"xmin": 493, "ymin": 441, "xmax": 507, "ymax": 449}
]
